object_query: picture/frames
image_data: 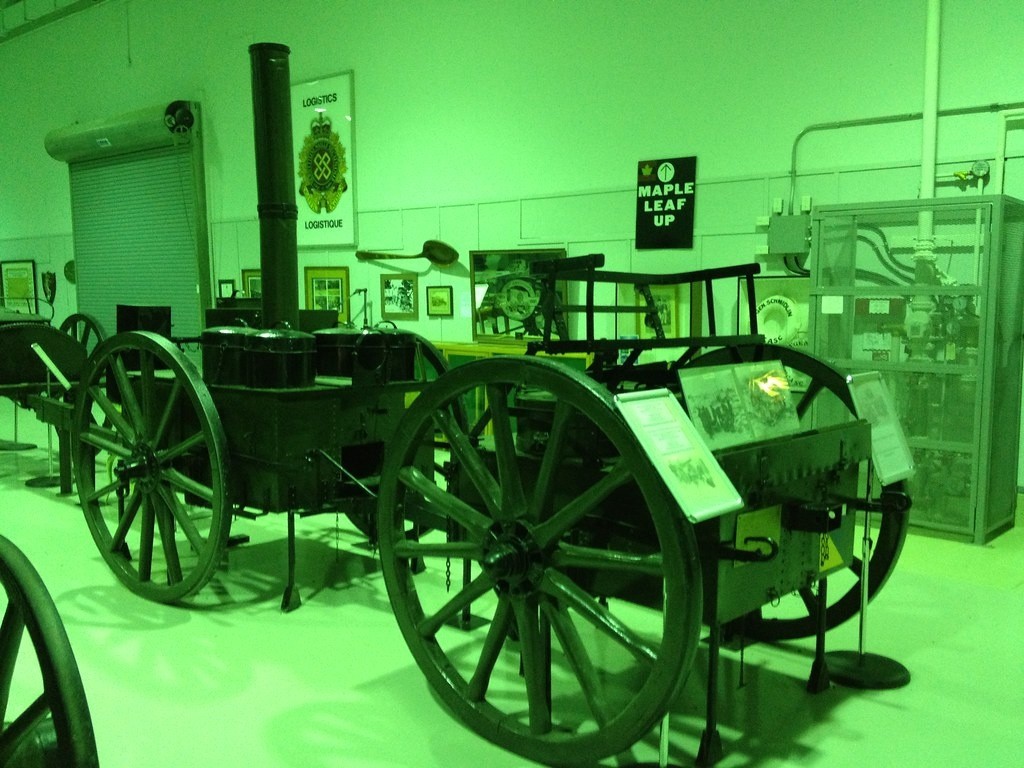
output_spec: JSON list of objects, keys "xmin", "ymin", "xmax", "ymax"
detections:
[
  {"xmin": 380, "ymin": 272, "xmax": 418, "ymax": 321},
  {"xmin": 241, "ymin": 269, "xmax": 261, "ymax": 297},
  {"xmin": 426, "ymin": 286, "xmax": 453, "ymax": 316},
  {"xmin": 0, "ymin": 260, "xmax": 38, "ymax": 313},
  {"xmin": 218, "ymin": 279, "xmax": 235, "ymax": 298},
  {"xmin": 304, "ymin": 266, "xmax": 350, "ymax": 325}
]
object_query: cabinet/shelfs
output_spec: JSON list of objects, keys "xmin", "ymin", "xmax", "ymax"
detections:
[{"xmin": 809, "ymin": 195, "xmax": 1024, "ymax": 544}]
